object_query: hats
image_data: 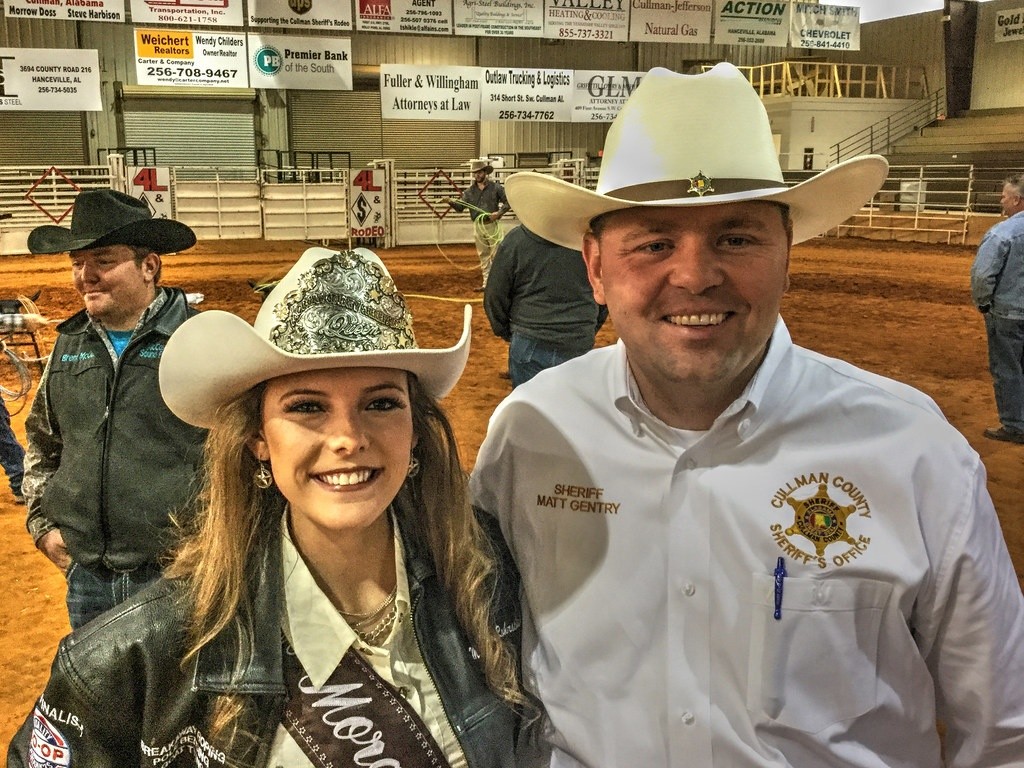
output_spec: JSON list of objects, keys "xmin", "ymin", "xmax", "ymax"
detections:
[
  {"xmin": 467, "ymin": 161, "xmax": 493, "ymax": 174},
  {"xmin": 27, "ymin": 189, "xmax": 197, "ymax": 254},
  {"xmin": 159, "ymin": 246, "xmax": 474, "ymax": 430},
  {"xmin": 504, "ymin": 62, "xmax": 890, "ymax": 251}
]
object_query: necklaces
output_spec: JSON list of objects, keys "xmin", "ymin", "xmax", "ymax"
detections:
[{"xmin": 330, "ymin": 581, "xmax": 398, "ymax": 646}]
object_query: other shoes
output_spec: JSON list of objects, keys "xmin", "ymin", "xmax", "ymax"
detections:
[
  {"xmin": 473, "ymin": 286, "xmax": 485, "ymax": 292},
  {"xmin": 983, "ymin": 425, "xmax": 1024, "ymax": 445}
]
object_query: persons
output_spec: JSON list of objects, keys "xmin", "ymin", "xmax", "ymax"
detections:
[
  {"xmin": 482, "ymin": 223, "xmax": 610, "ymax": 393},
  {"xmin": 467, "ymin": 61, "xmax": 1024, "ymax": 768},
  {"xmin": 19, "ymin": 187, "xmax": 209, "ymax": 636},
  {"xmin": 969, "ymin": 173, "xmax": 1024, "ymax": 445},
  {"xmin": 5, "ymin": 246, "xmax": 553, "ymax": 768},
  {"xmin": 442, "ymin": 158, "xmax": 511, "ymax": 293},
  {"xmin": 0, "ymin": 392, "xmax": 32, "ymax": 506}
]
